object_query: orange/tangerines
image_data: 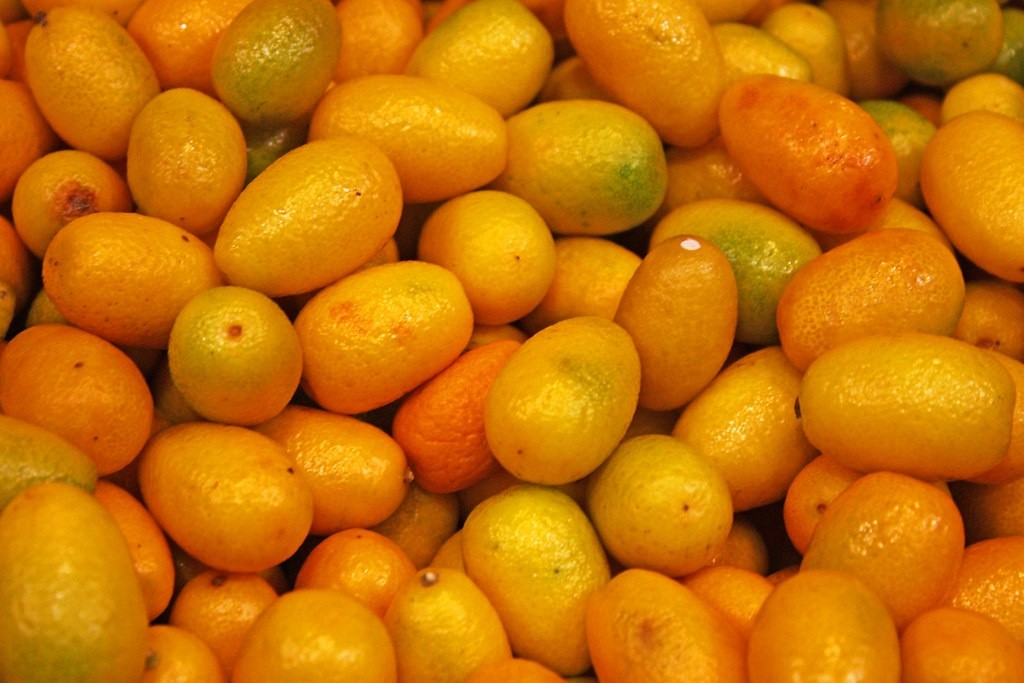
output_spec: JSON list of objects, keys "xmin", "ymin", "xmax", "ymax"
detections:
[{"xmin": 0, "ymin": 0, "xmax": 1024, "ymax": 683}]
